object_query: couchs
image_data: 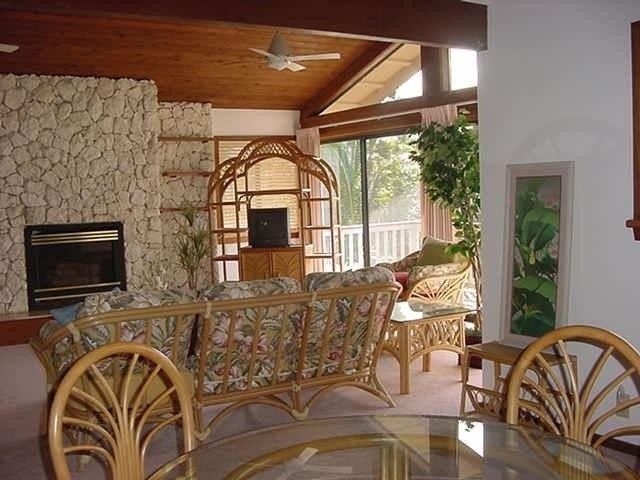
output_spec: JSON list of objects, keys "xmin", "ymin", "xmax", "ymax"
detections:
[{"xmin": 28, "ymin": 267, "xmax": 403, "ymax": 470}]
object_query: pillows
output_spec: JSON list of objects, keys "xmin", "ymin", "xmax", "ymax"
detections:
[
  {"xmin": 418, "ymin": 235, "xmax": 457, "ymax": 266},
  {"xmin": 49, "ymin": 301, "xmax": 80, "ymax": 324}
]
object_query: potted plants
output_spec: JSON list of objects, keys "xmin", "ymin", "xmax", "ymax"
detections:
[{"xmin": 405, "ymin": 106, "xmax": 482, "ymax": 370}]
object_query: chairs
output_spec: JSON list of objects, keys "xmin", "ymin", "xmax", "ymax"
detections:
[
  {"xmin": 46, "ymin": 341, "xmax": 197, "ymax": 479},
  {"xmin": 373, "ymin": 235, "xmax": 473, "ymax": 300},
  {"xmin": 505, "ymin": 323, "xmax": 640, "ymax": 480}
]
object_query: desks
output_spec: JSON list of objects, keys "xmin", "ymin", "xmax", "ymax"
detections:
[
  {"xmin": 0, "ymin": 310, "xmax": 55, "ymax": 346},
  {"xmin": 460, "ymin": 341, "xmax": 578, "ymax": 433}
]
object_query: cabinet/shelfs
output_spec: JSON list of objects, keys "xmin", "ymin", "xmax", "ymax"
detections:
[
  {"xmin": 238, "ymin": 248, "xmax": 305, "ymax": 280},
  {"xmin": 208, "ymin": 137, "xmax": 342, "ymax": 282}
]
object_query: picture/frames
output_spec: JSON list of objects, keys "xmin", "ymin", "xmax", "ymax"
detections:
[{"xmin": 499, "ymin": 160, "xmax": 575, "ymax": 358}]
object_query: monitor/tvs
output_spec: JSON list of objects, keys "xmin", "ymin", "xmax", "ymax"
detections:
[{"xmin": 246, "ymin": 207, "xmax": 289, "ymax": 248}]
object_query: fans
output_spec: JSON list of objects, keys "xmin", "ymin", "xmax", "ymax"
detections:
[{"xmin": 221, "ymin": 28, "xmax": 344, "ymax": 72}]
